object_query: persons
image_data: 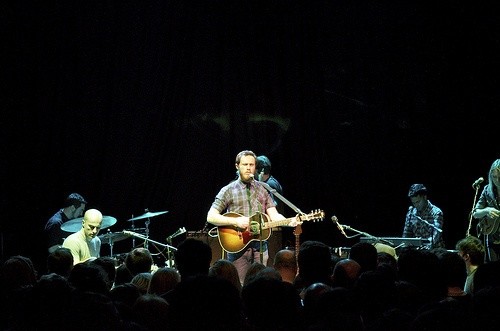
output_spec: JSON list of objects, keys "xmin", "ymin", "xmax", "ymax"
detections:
[
  {"xmin": 43, "ymin": 193, "xmax": 86, "ymax": 245},
  {"xmin": 0, "ymin": 237, "xmax": 500, "ymax": 331},
  {"xmin": 255, "ymin": 156, "xmax": 283, "ymax": 207},
  {"xmin": 207, "ymin": 150, "xmax": 303, "ymax": 287},
  {"xmin": 473, "ymin": 159, "xmax": 500, "ymax": 262},
  {"xmin": 402, "ymin": 183, "xmax": 445, "ymax": 248},
  {"xmin": 62, "ymin": 209, "xmax": 103, "ymax": 266}
]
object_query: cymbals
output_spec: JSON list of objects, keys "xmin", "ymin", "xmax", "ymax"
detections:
[
  {"xmin": 128, "ymin": 210, "xmax": 169, "ymax": 221},
  {"xmin": 60, "ymin": 215, "xmax": 117, "ymax": 233},
  {"xmin": 97, "ymin": 232, "xmax": 133, "ymax": 244}
]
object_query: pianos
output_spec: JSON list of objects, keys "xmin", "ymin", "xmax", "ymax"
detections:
[{"xmin": 359, "ymin": 236, "xmax": 431, "ymax": 247}]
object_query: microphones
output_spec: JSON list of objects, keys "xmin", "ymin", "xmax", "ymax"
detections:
[
  {"xmin": 408, "ymin": 205, "xmax": 414, "ymax": 215},
  {"xmin": 472, "ymin": 177, "xmax": 484, "ymax": 187},
  {"xmin": 249, "ymin": 173, "xmax": 255, "ymax": 182},
  {"xmin": 331, "ymin": 215, "xmax": 346, "ymax": 237}
]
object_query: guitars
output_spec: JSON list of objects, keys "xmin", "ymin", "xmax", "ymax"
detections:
[
  {"xmin": 479, "ymin": 214, "xmax": 500, "ymax": 235},
  {"xmin": 217, "ymin": 209, "xmax": 326, "ymax": 254}
]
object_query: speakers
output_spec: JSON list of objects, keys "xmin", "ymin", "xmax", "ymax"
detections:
[{"xmin": 186, "ymin": 231, "xmax": 229, "ymax": 272}]
